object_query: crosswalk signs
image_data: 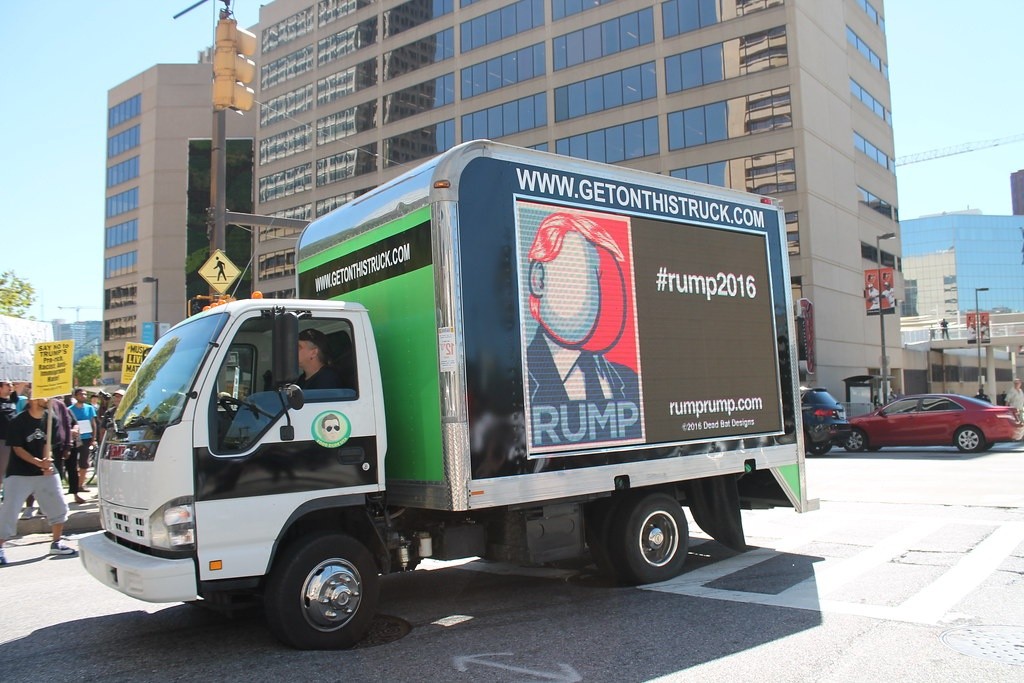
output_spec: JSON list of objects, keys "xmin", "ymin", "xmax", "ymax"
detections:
[{"xmin": 198, "ymin": 248, "xmax": 241, "ymax": 297}]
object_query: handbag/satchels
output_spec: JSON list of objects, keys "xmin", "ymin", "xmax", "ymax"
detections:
[{"xmin": 74, "ymin": 436, "xmax": 81, "ymax": 448}]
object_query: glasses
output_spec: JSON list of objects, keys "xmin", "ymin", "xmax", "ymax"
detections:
[{"xmin": 4, "ymin": 383, "xmax": 14, "ymax": 386}]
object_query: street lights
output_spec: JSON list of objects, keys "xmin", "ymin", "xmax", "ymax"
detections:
[
  {"xmin": 876, "ymin": 232, "xmax": 895, "ymax": 405},
  {"xmin": 975, "ymin": 288, "xmax": 989, "ymax": 394}
]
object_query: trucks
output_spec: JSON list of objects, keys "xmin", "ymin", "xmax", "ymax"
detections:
[{"xmin": 79, "ymin": 137, "xmax": 819, "ymax": 650}]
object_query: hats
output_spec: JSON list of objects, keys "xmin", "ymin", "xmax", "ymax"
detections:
[
  {"xmin": 113, "ymin": 389, "xmax": 126, "ymax": 396},
  {"xmin": 298, "ymin": 329, "xmax": 329, "ymax": 352}
]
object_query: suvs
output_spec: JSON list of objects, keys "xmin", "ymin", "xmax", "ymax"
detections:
[{"xmin": 799, "ymin": 385, "xmax": 852, "ymax": 455}]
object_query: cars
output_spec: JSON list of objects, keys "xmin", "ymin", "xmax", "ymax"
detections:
[{"xmin": 843, "ymin": 394, "xmax": 1024, "ymax": 453}]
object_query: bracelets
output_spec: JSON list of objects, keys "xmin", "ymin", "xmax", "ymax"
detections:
[{"xmin": 92, "ymin": 438, "xmax": 96, "ymax": 440}]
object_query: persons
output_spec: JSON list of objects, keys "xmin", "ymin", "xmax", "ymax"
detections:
[
  {"xmin": 889, "ymin": 389, "xmax": 905, "ymax": 403},
  {"xmin": 941, "ymin": 318, "xmax": 949, "ymax": 339},
  {"xmin": 974, "ymin": 378, "xmax": 1024, "ymax": 422},
  {"xmin": 980, "ymin": 315, "xmax": 989, "ymax": 339},
  {"xmin": 929, "ymin": 324, "xmax": 936, "ymax": 339},
  {"xmin": 966, "ymin": 316, "xmax": 976, "ymax": 338},
  {"xmin": 0, "ymin": 380, "xmax": 126, "ymax": 566},
  {"xmin": 124, "ymin": 445, "xmax": 150, "ymax": 461},
  {"xmin": 866, "ymin": 282, "xmax": 896, "ymax": 309},
  {"xmin": 217, "ymin": 328, "xmax": 346, "ymax": 400}
]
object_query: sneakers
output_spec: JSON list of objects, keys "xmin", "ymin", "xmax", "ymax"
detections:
[
  {"xmin": 0, "ymin": 550, "xmax": 6, "ymax": 564},
  {"xmin": 50, "ymin": 542, "xmax": 75, "ymax": 554}
]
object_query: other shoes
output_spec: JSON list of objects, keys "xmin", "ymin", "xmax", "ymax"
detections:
[
  {"xmin": 37, "ymin": 508, "xmax": 44, "ymax": 516},
  {"xmin": 75, "ymin": 495, "xmax": 86, "ymax": 503},
  {"xmin": 78, "ymin": 485, "xmax": 89, "ymax": 492},
  {"xmin": 20, "ymin": 507, "xmax": 32, "ymax": 519}
]
